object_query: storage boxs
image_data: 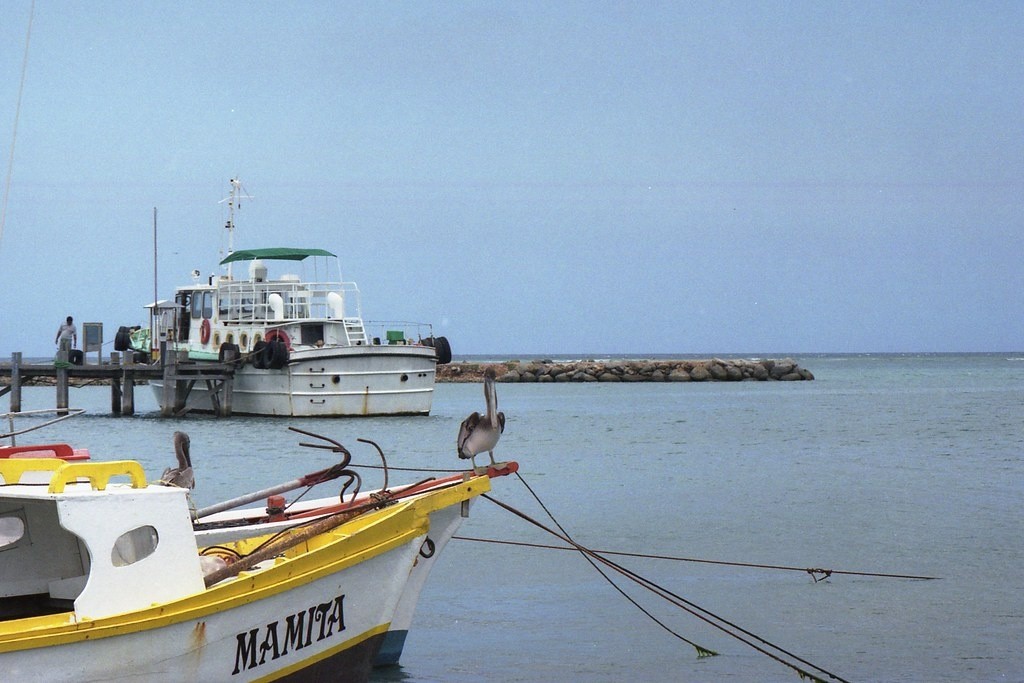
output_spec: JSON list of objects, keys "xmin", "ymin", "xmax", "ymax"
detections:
[{"xmin": 387, "ymin": 331, "xmax": 404, "ymax": 346}]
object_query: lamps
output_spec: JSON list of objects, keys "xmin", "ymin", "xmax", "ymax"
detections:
[{"xmin": 191, "ymin": 269, "xmax": 201, "ymax": 287}]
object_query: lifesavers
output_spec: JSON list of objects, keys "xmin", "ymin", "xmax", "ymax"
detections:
[
  {"xmin": 69, "ymin": 348, "xmax": 84, "ymax": 366},
  {"xmin": 218, "ymin": 342, "xmax": 240, "ymax": 366},
  {"xmin": 265, "ymin": 330, "xmax": 291, "ymax": 351},
  {"xmin": 263, "ymin": 341, "xmax": 287, "ymax": 370},
  {"xmin": 252, "ymin": 340, "xmax": 267, "ymax": 369},
  {"xmin": 200, "ymin": 319, "xmax": 210, "ymax": 345}
]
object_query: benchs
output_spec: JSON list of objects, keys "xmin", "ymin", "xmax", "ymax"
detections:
[{"xmin": 47, "ymin": 574, "xmax": 91, "ymax": 609}]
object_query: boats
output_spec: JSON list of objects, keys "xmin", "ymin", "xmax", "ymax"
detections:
[
  {"xmin": 1, "ymin": 399, "xmax": 522, "ymax": 683},
  {"xmin": 142, "ymin": 169, "xmax": 438, "ymax": 419}
]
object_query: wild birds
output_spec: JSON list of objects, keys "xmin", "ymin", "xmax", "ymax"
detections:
[
  {"xmin": 160, "ymin": 431, "xmax": 195, "ymax": 499},
  {"xmin": 457, "ymin": 367, "xmax": 508, "ymax": 477}
]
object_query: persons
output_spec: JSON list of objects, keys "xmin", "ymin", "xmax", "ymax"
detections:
[
  {"xmin": 55, "ymin": 316, "xmax": 77, "ymax": 363},
  {"xmin": 313, "ymin": 338, "xmax": 325, "ymax": 348}
]
